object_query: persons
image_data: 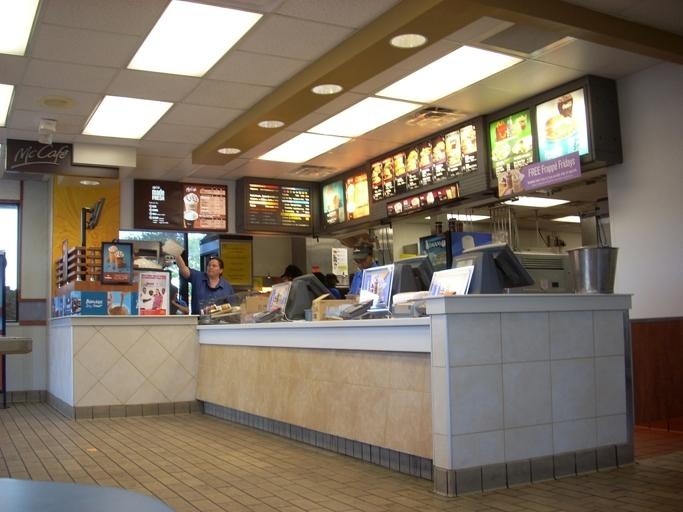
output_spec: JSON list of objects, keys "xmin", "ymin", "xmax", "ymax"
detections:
[
  {"xmin": 323, "ymin": 272, "xmax": 341, "ymax": 300},
  {"xmin": 139, "ymin": 286, "xmax": 148, "ymax": 307},
  {"xmin": 142, "ymin": 289, "xmax": 155, "ymax": 310},
  {"xmin": 170, "ymin": 284, "xmax": 189, "ymax": 315},
  {"xmin": 280, "ymin": 264, "xmax": 302, "ymax": 282},
  {"xmin": 160, "ymin": 288, "xmax": 167, "ymax": 310},
  {"xmin": 174, "ymin": 254, "xmax": 237, "ymax": 315},
  {"xmin": 152, "ymin": 288, "xmax": 162, "ymax": 310},
  {"xmin": 348, "ymin": 241, "xmax": 378, "ymax": 296}
]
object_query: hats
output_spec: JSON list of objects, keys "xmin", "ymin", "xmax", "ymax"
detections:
[
  {"xmin": 352, "ymin": 246, "xmax": 372, "ymax": 261},
  {"xmin": 280, "ymin": 265, "xmax": 301, "ymax": 278}
]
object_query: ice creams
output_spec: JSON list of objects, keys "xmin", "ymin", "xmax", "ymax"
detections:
[
  {"xmin": 114, "ymin": 251, "xmax": 124, "ymax": 269},
  {"xmin": 108, "ymin": 245, "xmax": 118, "ymax": 264},
  {"xmin": 183, "ymin": 192, "xmax": 199, "ymax": 213}
]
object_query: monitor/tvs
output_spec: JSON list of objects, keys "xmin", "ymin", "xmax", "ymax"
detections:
[
  {"xmin": 462, "ymin": 240, "xmax": 537, "ymax": 289},
  {"xmin": 294, "ymin": 273, "xmax": 340, "ymax": 301},
  {"xmin": 394, "ymin": 253, "xmax": 435, "ymax": 290}
]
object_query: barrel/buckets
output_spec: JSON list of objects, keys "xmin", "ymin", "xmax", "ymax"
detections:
[{"xmin": 566, "ymin": 245, "xmax": 619, "ymax": 295}]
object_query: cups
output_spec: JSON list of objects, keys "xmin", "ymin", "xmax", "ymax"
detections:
[{"xmin": 556, "ymin": 93, "xmax": 574, "ymax": 117}]
